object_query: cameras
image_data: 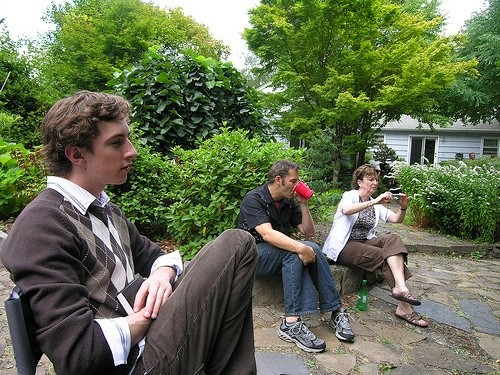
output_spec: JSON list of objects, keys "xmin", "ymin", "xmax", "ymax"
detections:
[{"xmin": 389, "ymin": 188, "xmax": 402, "ymax": 200}]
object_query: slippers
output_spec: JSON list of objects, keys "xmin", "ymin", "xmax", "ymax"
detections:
[
  {"xmin": 394, "ymin": 311, "xmax": 428, "ymax": 328},
  {"xmin": 392, "ymin": 289, "xmax": 421, "ymax": 306}
]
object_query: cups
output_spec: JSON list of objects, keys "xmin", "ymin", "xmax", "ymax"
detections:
[{"xmin": 293, "ymin": 181, "xmax": 314, "ymax": 199}]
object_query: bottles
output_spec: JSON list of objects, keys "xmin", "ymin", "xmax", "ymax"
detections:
[{"xmin": 357, "ymin": 279, "xmax": 369, "ymax": 311}]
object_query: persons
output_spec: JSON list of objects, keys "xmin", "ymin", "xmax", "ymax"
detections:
[
  {"xmin": 0, "ymin": 90, "xmax": 260, "ymax": 375},
  {"xmin": 237, "ymin": 159, "xmax": 356, "ymax": 353},
  {"xmin": 321, "ymin": 164, "xmax": 428, "ymax": 328},
  {"xmin": 469, "ymin": 153, "xmax": 475, "ymax": 160}
]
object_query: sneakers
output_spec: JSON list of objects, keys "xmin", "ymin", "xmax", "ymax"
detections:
[
  {"xmin": 328, "ymin": 310, "xmax": 354, "ymax": 341},
  {"xmin": 278, "ymin": 319, "xmax": 326, "ymax": 352}
]
